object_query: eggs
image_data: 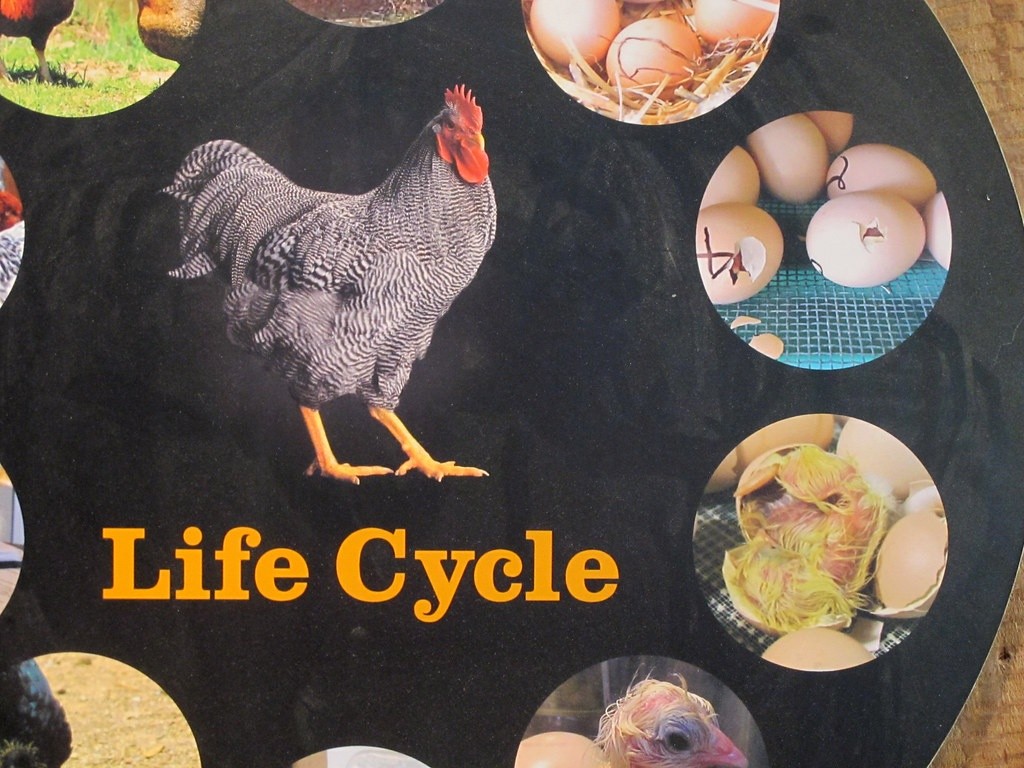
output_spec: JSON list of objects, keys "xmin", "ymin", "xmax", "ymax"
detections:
[
  {"xmin": 528, "ymin": 0, "xmax": 952, "ymax": 306},
  {"xmin": 703, "ymin": 414, "xmax": 950, "ymax": 671}
]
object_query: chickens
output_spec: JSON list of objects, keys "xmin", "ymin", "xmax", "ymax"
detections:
[
  {"xmin": 156, "ymin": 77, "xmax": 496, "ymax": 489},
  {"xmin": 515, "ymin": 660, "xmax": 750, "ymax": 768}
]
